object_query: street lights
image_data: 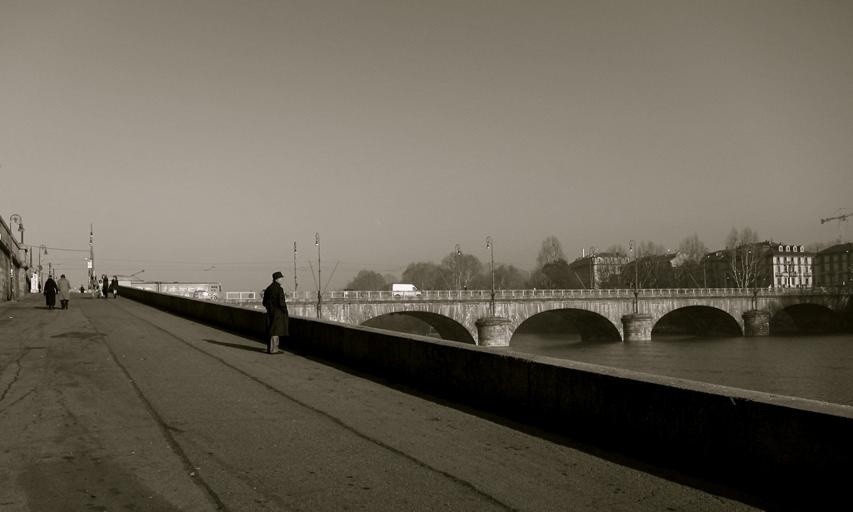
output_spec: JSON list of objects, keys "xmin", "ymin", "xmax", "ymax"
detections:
[
  {"xmin": 454, "ymin": 243, "xmax": 461, "ymax": 289},
  {"xmin": 627, "ymin": 239, "xmax": 638, "ymax": 313},
  {"xmin": 314, "ymin": 231, "xmax": 322, "ymax": 318},
  {"xmin": 485, "ymin": 236, "xmax": 496, "ymax": 316},
  {"xmin": 292, "ymin": 239, "xmax": 298, "ymax": 290},
  {"xmin": 37, "ymin": 242, "xmax": 48, "ymax": 291},
  {"xmin": 8, "ymin": 212, "xmax": 24, "ymax": 304}
]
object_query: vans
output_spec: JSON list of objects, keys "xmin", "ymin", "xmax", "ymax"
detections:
[{"xmin": 390, "ymin": 283, "xmax": 422, "ymax": 298}]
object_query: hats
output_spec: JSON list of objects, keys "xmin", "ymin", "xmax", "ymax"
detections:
[{"xmin": 272, "ymin": 271, "xmax": 284, "ymax": 281}]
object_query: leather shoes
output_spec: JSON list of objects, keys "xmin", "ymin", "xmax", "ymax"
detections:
[{"xmin": 267, "ymin": 348, "xmax": 284, "ymax": 354}]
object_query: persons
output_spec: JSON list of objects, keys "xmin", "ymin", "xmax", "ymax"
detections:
[
  {"xmin": 79, "ymin": 285, "xmax": 85, "ymax": 293},
  {"xmin": 98, "ymin": 280, "xmax": 104, "ymax": 298},
  {"xmin": 102, "ymin": 274, "xmax": 108, "ymax": 298},
  {"xmin": 111, "ymin": 276, "xmax": 119, "ymax": 298},
  {"xmin": 44, "ymin": 275, "xmax": 59, "ymax": 310},
  {"xmin": 57, "ymin": 273, "xmax": 71, "ymax": 310},
  {"xmin": 263, "ymin": 271, "xmax": 290, "ymax": 354}
]
